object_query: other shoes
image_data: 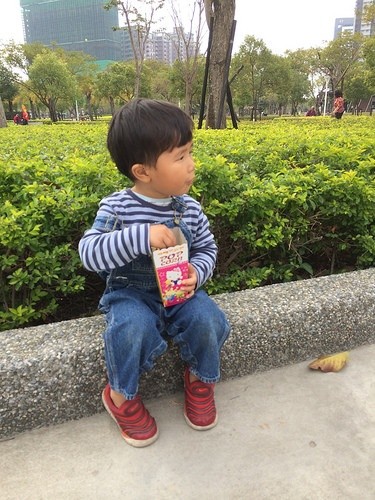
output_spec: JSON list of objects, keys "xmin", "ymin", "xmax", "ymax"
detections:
[
  {"xmin": 102, "ymin": 382, "xmax": 158, "ymax": 447},
  {"xmin": 183, "ymin": 368, "xmax": 217, "ymax": 430}
]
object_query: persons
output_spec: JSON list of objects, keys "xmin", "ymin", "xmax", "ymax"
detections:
[
  {"xmin": 14, "ymin": 113, "xmax": 29, "ymax": 125},
  {"xmin": 333, "ymin": 90, "xmax": 344, "ymax": 120},
  {"xmin": 78, "ymin": 97, "xmax": 230, "ymax": 447},
  {"xmin": 306, "ymin": 106, "xmax": 315, "ymax": 116}
]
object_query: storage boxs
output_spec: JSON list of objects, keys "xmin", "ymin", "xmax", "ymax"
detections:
[{"xmin": 149, "ymin": 226, "xmax": 190, "ymax": 307}]
